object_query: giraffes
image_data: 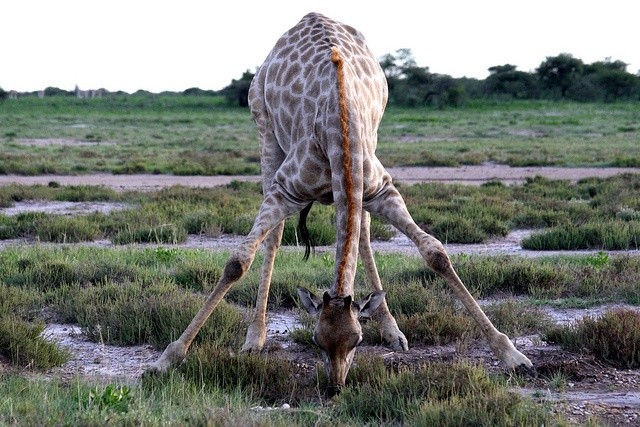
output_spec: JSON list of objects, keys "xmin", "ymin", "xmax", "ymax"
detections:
[{"xmin": 139, "ymin": 11, "xmax": 541, "ymax": 400}]
